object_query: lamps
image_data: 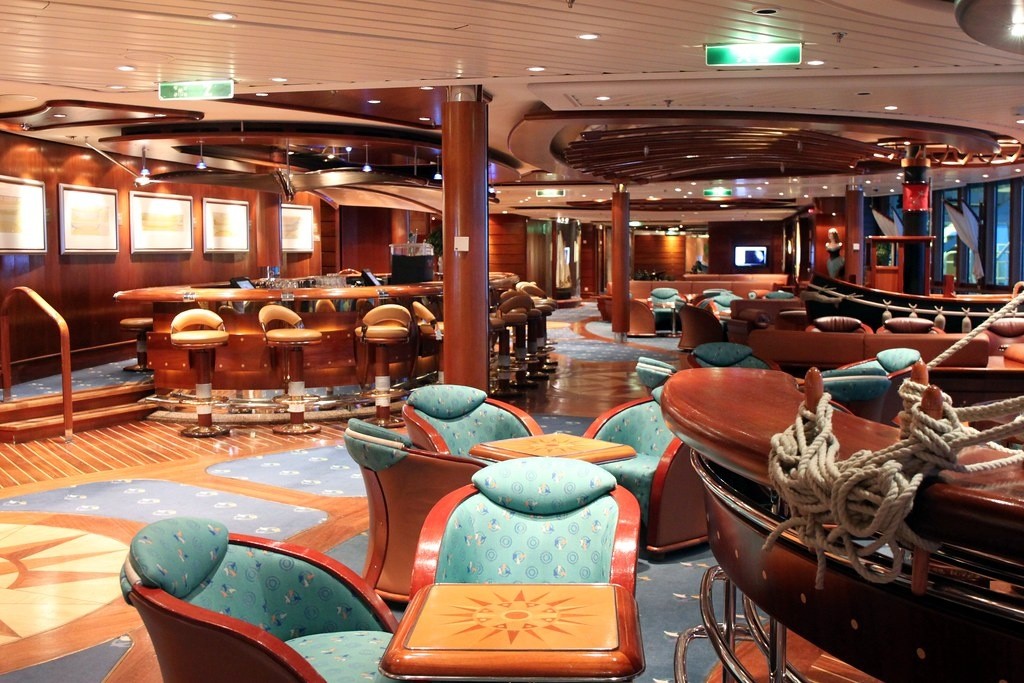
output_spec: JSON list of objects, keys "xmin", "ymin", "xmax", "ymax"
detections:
[
  {"xmin": 433, "ymin": 156, "xmax": 443, "ymax": 180},
  {"xmin": 139, "ymin": 148, "xmax": 149, "ymax": 175},
  {"xmin": 363, "ymin": 145, "xmax": 372, "ymax": 172},
  {"xmin": 197, "ymin": 141, "xmax": 207, "ymax": 169}
]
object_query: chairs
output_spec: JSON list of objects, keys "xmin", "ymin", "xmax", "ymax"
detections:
[
  {"xmin": 344, "ymin": 418, "xmax": 490, "ymax": 603},
  {"xmin": 583, "ymin": 395, "xmax": 704, "ymax": 562},
  {"xmin": 402, "ymin": 385, "xmax": 541, "ymax": 455},
  {"xmin": 121, "ymin": 516, "xmax": 400, "ymax": 683},
  {"xmin": 636, "ymin": 288, "xmax": 1024, "ymax": 683}
]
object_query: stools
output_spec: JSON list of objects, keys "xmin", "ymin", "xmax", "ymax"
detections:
[{"xmin": 118, "ymin": 283, "xmax": 558, "ymax": 438}]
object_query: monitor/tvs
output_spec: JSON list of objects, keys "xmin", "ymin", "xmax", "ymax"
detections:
[
  {"xmin": 733, "ymin": 244, "xmax": 768, "ymax": 268},
  {"xmin": 361, "ymin": 268, "xmax": 382, "ymax": 286},
  {"xmin": 230, "ymin": 276, "xmax": 257, "ymax": 290}
]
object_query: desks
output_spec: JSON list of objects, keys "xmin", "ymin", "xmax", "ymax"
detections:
[
  {"xmin": 470, "ymin": 432, "xmax": 637, "ymax": 466},
  {"xmin": 381, "ymin": 582, "xmax": 644, "ymax": 682}
]
